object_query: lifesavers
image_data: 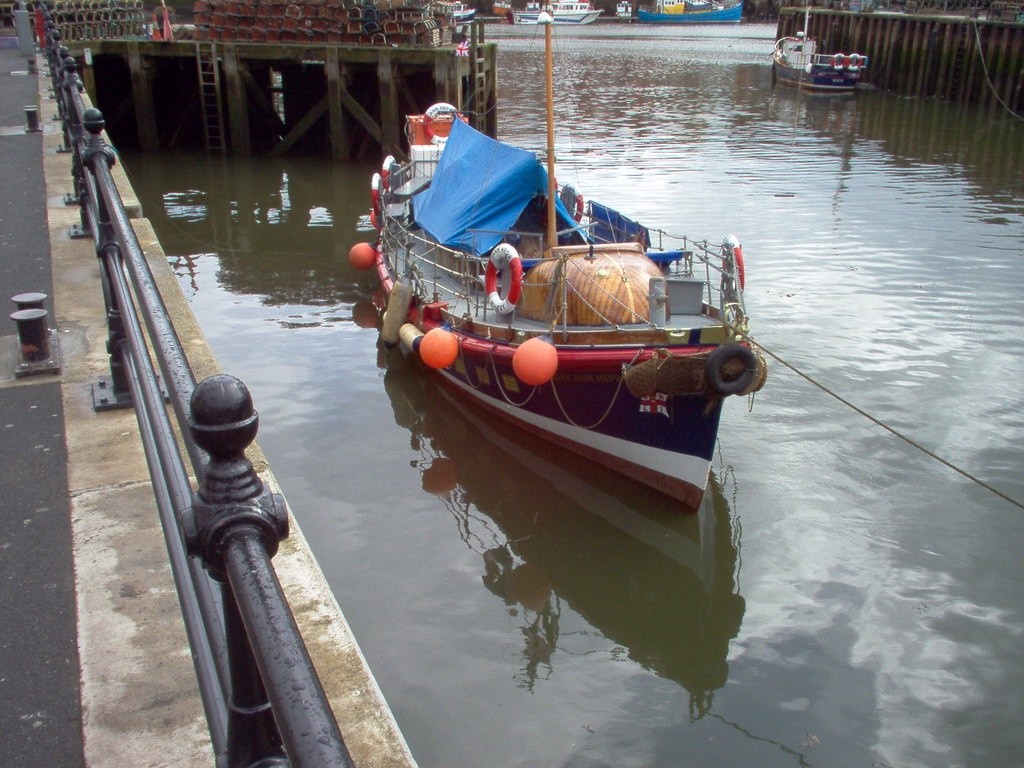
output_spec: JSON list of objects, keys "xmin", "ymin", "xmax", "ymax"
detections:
[
  {"xmin": 847, "ymin": 53, "xmax": 863, "ymax": 71},
  {"xmin": 705, "ymin": 342, "xmax": 757, "ymax": 394},
  {"xmin": 372, "ymin": 172, "xmax": 383, "ymax": 215},
  {"xmin": 573, "ymin": 194, "xmax": 584, "ymax": 222},
  {"xmin": 382, "ymin": 155, "xmax": 396, "ymax": 191},
  {"xmin": 722, "ymin": 233, "xmax": 747, "ymax": 305},
  {"xmin": 485, "ymin": 242, "xmax": 522, "ymax": 315},
  {"xmin": 832, "ymin": 53, "xmax": 845, "ymax": 71},
  {"xmin": 423, "ymin": 103, "xmax": 464, "ymax": 146}
]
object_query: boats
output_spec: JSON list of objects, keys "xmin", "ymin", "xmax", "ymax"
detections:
[
  {"xmin": 637, "ymin": 0, "xmax": 743, "ymax": 29},
  {"xmin": 372, "ymin": 101, "xmax": 768, "ymax": 515},
  {"xmin": 443, "ymin": 1, "xmax": 477, "ymax": 22},
  {"xmin": 516, "ymin": 1, "xmax": 604, "ymax": 25},
  {"xmin": 373, "ymin": 324, "xmax": 747, "ymax": 694},
  {"xmin": 615, "ymin": 1, "xmax": 638, "ymax": 20},
  {"xmin": 769, "ymin": 9, "xmax": 869, "ymax": 93}
]
